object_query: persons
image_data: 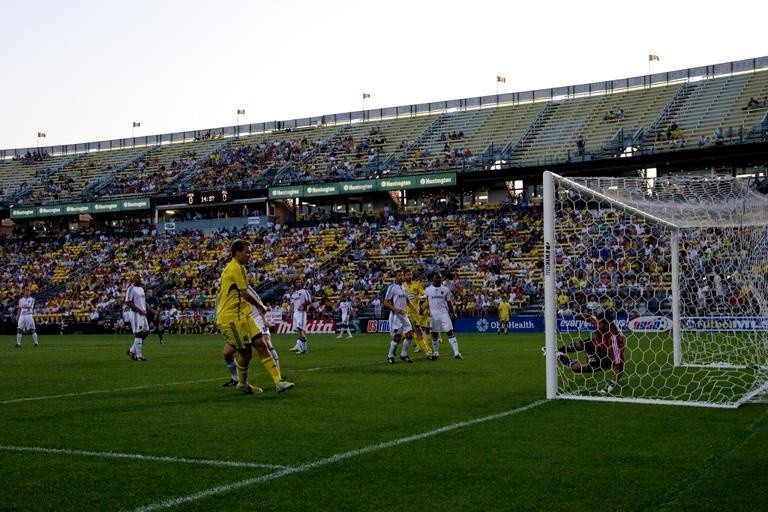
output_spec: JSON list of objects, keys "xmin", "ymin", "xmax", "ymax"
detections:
[
  {"xmin": 384, "ymin": 270, "xmax": 463, "ymax": 364},
  {"xmin": 16, "ymin": 287, "xmax": 38, "ymax": 348},
  {"xmin": 680, "ymin": 166, "xmax": 768, "ymax": 201},
  {"xmin": 742, "ymin": 98, "xmax": 767, "ymax": 110},
  {"xmin": 541, "ymin": 312, "xmax": 625, "ymax": 394},
  {"xmin": 125, "ymin": 275, "xmax": 165, "ymax": 361},
  {"xmin": 2, "ymin": 97, "xmax": 685, "ymax": 202},
  {"xmin": 215, "ymin": 239, "xmax": 296, "ymax": 393},
  {"xmin": 287, "ymin": 278, "xmax": 311, "ymax": 355},
  {"xmin": 698, "ymin": 123, "xmax": 745, "ymax": 146},
  {"xmin": 2, "ymin": 193, "xmax": 768, "ymax": 339}
]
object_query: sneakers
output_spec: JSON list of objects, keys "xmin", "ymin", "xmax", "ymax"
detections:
[
  {"xmin": 33, "ymin": 342, "xmax": 38, "ymax": 347},
  {"xmin": 414, "ymin": 346, "xmax": 439, "ymax": 360},
  {"xmin": 541, "ymin": 346, "xmax": 563, "ymax": 359},
  {"xmin": 222, "ymin": 378, "xmax": 239, "ymax": 387},
  {"xmin": 276, "ymin": 380, "xmax": 295, "ymax": 393},
  {"xmin": 387, "ymin": 355, "xmax": 394, "ymax": 364},
  {"xmin": 15, "ymin": 343, "xmax": 21, "ymax": 348},
  {"xmin": 400, "ymin": 355, "xmax": 411, "ymax": 362},
  {"xmin": 127, "ymin": 348, "xmax": 147, "ymax": 361},
  {"xmin": 290, "ymin": 346, "xmax": 307, "ymax": 354},
  {"xmin": 236, "ymin": 383, "xmax": 263, "ymax": 394},
  {"xmin": 336, "ymin": 336, "xmax": 353, "ymax": 339},
  {"xmin": 455, "ymin": 352, "xmax": 464, "ymax": 360}
]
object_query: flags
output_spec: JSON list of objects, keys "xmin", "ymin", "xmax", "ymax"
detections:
[
  {"xmin": 363, "ymin": 93, "xmax": 370, "ymax": 99},
  {"xmin": 497, "ymin": 76, "xmax": 506, "ymax": 83},
  {"xmin": 649, "ymin": 55, "xmax": 659, "ymax": 61}
]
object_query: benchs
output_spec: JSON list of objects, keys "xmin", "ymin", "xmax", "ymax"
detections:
[{"xmin": 0, "ymin": 68, "xmax": 768, "ymax": 314}]
